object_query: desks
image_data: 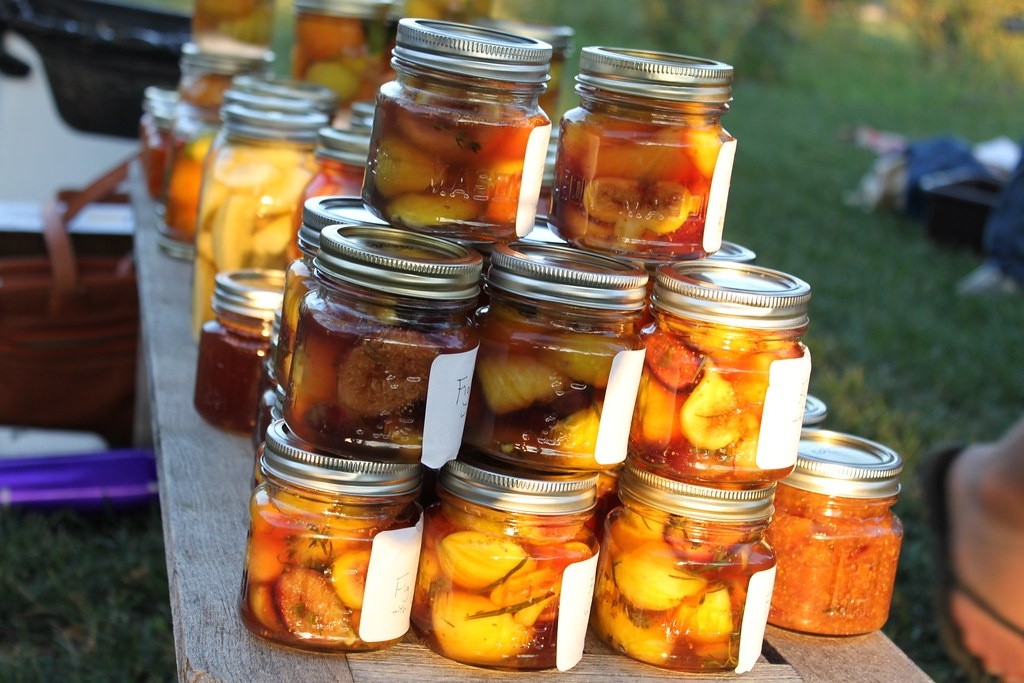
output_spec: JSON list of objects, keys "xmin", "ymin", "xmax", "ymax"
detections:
[{"xmin": 127, "ymin": 154, "xmax": 937, "ymax": 683}]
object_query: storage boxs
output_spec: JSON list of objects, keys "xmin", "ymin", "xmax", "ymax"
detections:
[{"xmin": 919, "ymin": 164, "xmax": 1011, "ymax": 250}]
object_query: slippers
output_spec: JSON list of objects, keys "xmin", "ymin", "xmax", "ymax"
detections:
[{"xmin": 917, "ymin": 443, "xmax": 1024, "ymax": 683}]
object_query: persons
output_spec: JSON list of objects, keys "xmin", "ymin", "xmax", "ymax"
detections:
[{"xmin": 918, "ymin": 415, "xmax": 1024, "ymax": 683}]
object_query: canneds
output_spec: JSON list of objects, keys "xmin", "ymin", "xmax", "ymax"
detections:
[{"xmin": 145, "ymin": 0, "xmax": 903, "ymax": 676}]
